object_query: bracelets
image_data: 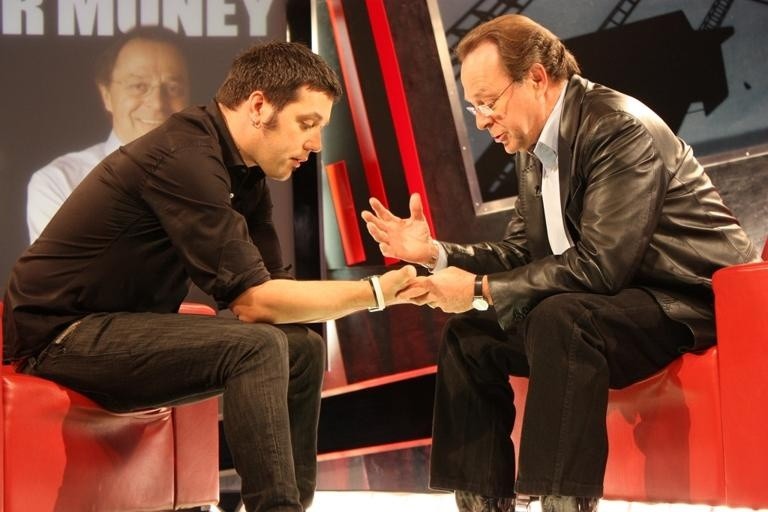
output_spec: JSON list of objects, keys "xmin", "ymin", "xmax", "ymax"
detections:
[{"xmin": 361, "ymin": 273, "xmax": 388, "ymax": 314}]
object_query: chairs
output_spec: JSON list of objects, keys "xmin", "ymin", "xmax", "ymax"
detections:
[
  {"xmin": 0, "ymin": 296, "xmax": 218, "ymax": 511},
  {"xmin": 505, "ymin": 237, "xmax": 766, "ymax": 512}
]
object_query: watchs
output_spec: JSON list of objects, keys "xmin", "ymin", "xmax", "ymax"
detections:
[{"xmin": 471, "ymin": 272, "xmax": 490, "ymax": 312}]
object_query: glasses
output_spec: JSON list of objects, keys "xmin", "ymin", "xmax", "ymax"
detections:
[{"xmin": 465, "ymin": 77, "xmax": 518, "ymax": 117}]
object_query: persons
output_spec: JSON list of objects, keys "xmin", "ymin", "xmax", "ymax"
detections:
[
  {"xmin": 24, "ymin": 24, "xmax": 192, "ymax": 249},
  {"xmin": 357, "ymin": 12, "xmax": 757, "ymax": 512},
  {"xmin": 1, "ymin": 41, "xmax": 419, "ymax": 511}
]
